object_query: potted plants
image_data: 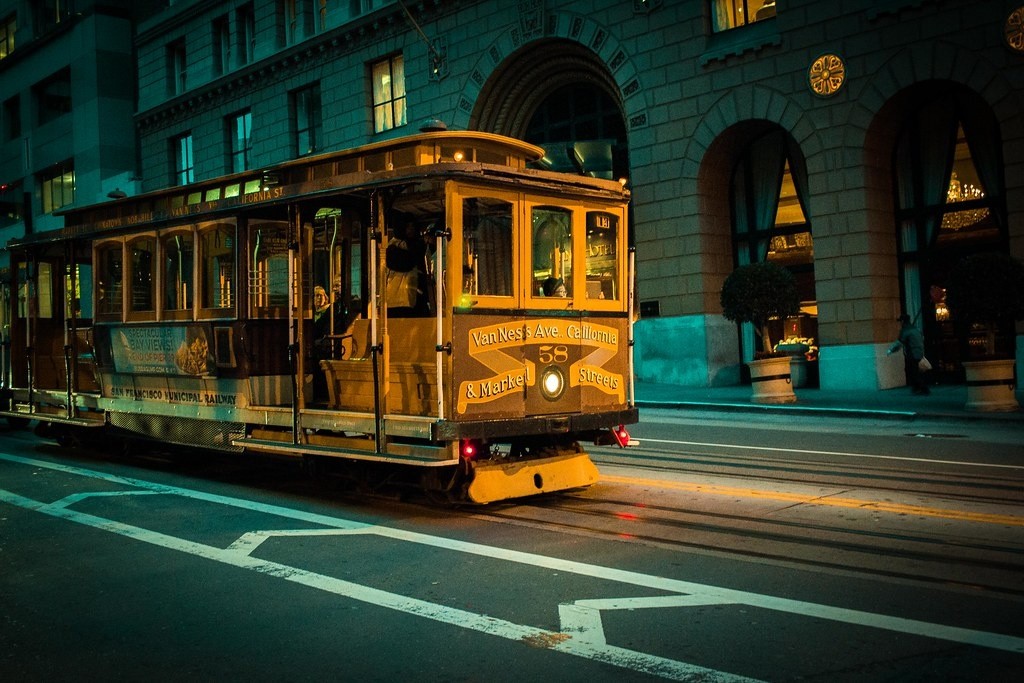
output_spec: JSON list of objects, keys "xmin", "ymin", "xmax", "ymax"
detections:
[{"xmin": 720, "ymin": 258, "xmax": 801, "ymax": 406}]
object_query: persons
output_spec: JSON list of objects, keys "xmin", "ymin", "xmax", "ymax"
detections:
[
  {"xmin": 887, "ymin": 315, "xmax": 934, "ymax": 398},
  {"xmin": 313, "ymin": 273, "xmax": 344, "ymax": 337},
  {"xmin": 542, "ymin": 278, "xmax": 567, "ymax": 298},
  {"xmin": 462, "ymin": 265, "xmax": 474, "ymax": 291},
  {"xmin": 378, "ymin": 215, "xmax": 429, "ymax": 314}
]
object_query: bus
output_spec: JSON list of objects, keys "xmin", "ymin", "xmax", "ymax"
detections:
[{"xmin": 1, "ymin": 118, "xmax": 641, "ymax": 512}]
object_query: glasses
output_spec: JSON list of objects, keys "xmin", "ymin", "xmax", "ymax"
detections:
[{"xmin": 554, "ymin": 290, "xmax": 568, "ymax": 294}]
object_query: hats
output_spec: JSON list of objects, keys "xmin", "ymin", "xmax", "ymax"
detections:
[{"xmin": 543, "ymin": 277, "xmax": 563, "ymax": 296}]
